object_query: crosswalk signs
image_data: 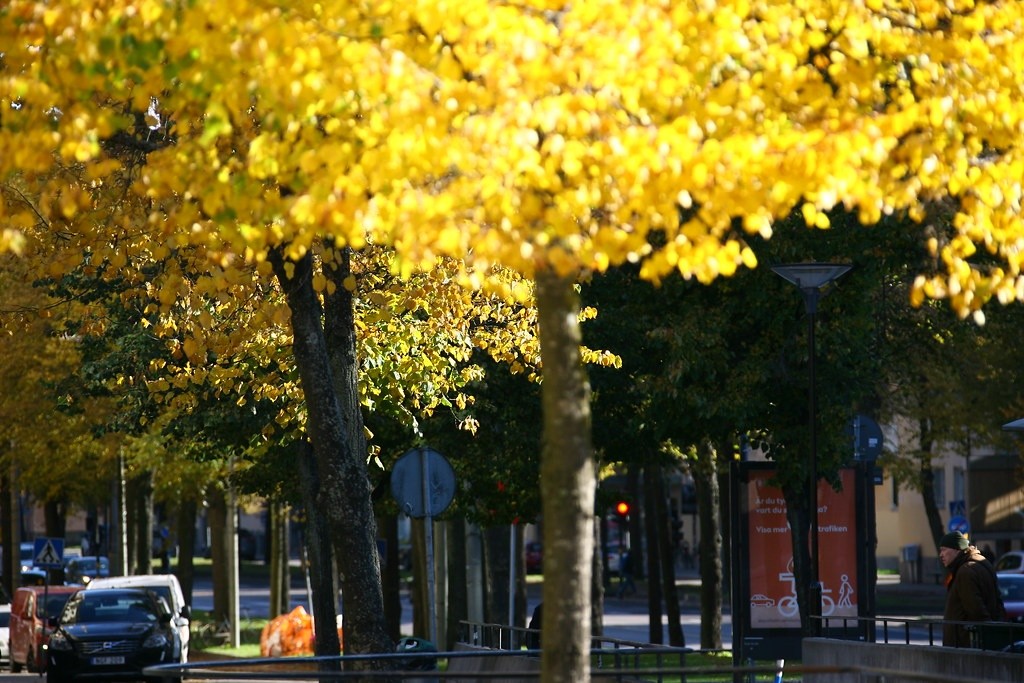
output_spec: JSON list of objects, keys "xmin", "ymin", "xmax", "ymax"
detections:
[{"xmin": 31, "ymin": 538, "xmax": 64, "ymax": 569}]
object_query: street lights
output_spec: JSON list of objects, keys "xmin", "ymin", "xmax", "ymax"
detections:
[{"xmin": 765, "ymin": 262, "xmax": 854, "ymax": 636}]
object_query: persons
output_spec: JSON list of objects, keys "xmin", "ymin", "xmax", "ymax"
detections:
[
  {"xmin": 939, "ymin": 531, "xmax": 1012, "ymax": 651},
  {"xmin": 526, "ymin": 598, "xmax": 542, "ymax": 649}
]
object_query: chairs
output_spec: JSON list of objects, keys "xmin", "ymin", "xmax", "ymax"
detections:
[
  {"xmin": 128, "ymin": 604, "xmax": 153, "ymax": 618},
  {"xmin": 79, "ymin": 606, "xmax": 97, "ymax": 621}
]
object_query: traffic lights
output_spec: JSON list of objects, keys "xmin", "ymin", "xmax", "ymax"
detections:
[
  {"xmin": 612, "ymin": 502, "xmax": 630, "ymax": 533},
  {"xmin": 671, "ymin": 509, "xmax": 684, "ymax": 547}
]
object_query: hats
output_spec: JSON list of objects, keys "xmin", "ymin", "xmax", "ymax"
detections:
[{"xmin": 939, "ymin": 531, "xmax": 969, "ymax": 550}]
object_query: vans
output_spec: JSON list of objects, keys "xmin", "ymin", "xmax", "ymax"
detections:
[
  {"xmin": 85, "ymin": 574, "xmax": 193, "ymax": 683},
  {"xmin": 8, "ymin": 585, "xmax": 85, "ymax": 673}
]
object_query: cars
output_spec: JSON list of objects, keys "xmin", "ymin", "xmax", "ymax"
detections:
[
  {"xmin": 525, "ymin": 542, "xmax": 544, "ymax": 575},
  {"xmin": 997, "ymin": 573, "xmax": 1024, "ymax": 624},
  {"xmin": 0, "ymin": 604, "xmax": 12, "ymax": 664},
  {"xmin": 62, "ymin": 555, "xmax": 109, "ymax": 586},
  {"xmin": 607, "ymin": 541, "xmax": 628, "ymax": 574},
  {"xmin": 45, "ymin": 588, "xmax": 189, "ymax": 683},
  {"xmin": 994, "ymin": 553, "xmax": 1024, "ymax": 574},
  {"xmin": 19, "ymin": 540, "xmax": 80, "ymax": 584}
]
